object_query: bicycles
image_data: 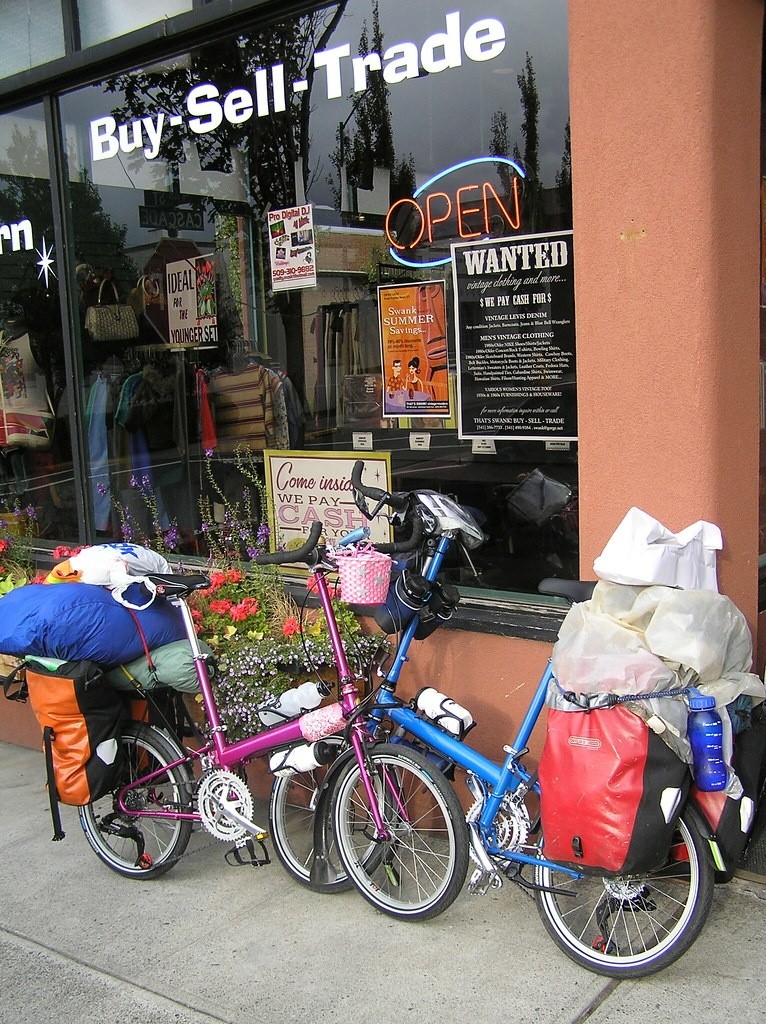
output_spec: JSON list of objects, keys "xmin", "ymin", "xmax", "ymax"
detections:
[
  {"xmin": 75, "ymin": 520, "xmax": 471, "ymax": 923},
  {"xmin": 266, "ymin": 458, "xmax": 725, "ymax": 980}
]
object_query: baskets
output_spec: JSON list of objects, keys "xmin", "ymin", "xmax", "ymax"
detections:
[{"xmin": 332, "ymin": 542, "xmax": 392, "ymax": 604}]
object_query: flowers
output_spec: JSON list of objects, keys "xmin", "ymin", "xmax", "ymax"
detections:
[
  {"xmin": 53, "ymin": 441, "xmax": 392, "ymax": 749},
  {"xmin": 0, "ymin": 493, "xmax": 53, "ymax": 596}
]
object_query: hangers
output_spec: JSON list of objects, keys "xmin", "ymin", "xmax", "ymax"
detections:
[
  {"xmin": 219, "ymin": 339, "xmax": 282, "ymax": 372},
  {"xmin": 121, "ymin": 344, "xmax": 203, "ymax": 375}
]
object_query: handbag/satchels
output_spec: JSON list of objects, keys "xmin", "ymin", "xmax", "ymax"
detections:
[
  {"xmin": 127, "ymin": 274, "xmax": 169, "ymax": 322},
  {"xmin": 128, "ymin": 686, "xmax": 178, "ymax": 785},
  {"xmin": 84, "ymin": 278, "xmax": 140, "ymax": 342},
  {"xmin": 3, "ymin": 655, "xmax": 125, "ymax": 807},
  {"xmin": 69, "ymin": 542, "xmax": 173, "ymax": 611},
  {"xmin": 3, "ymin": 406, "xmax": 57, "ymax": 451},
  {"xmin": 538, "ymin": 646, "xmax": 693, "ymax": 876},
  {"xmin": 649, "ymin": 703, "xmax": 765, "ymax": 886}
]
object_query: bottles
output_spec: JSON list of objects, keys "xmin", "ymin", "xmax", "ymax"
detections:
[
  {"xmin": 268, "ymin": 742, "xmax": 340, "ymax": 779},
  {"xmin": 256, "ymin": 682, "xmax": 337, "ymax": 727},
  {"xmin": 372, "ymin": 575, "xmax": 460, "ymax": 644},
  {"xmin": 684, "ymin": 695, "xmax": 728, "ymax": 791},
  {"xmin": 415, "ymin": 688, "xmax": 473, "ymax": 736}
]
